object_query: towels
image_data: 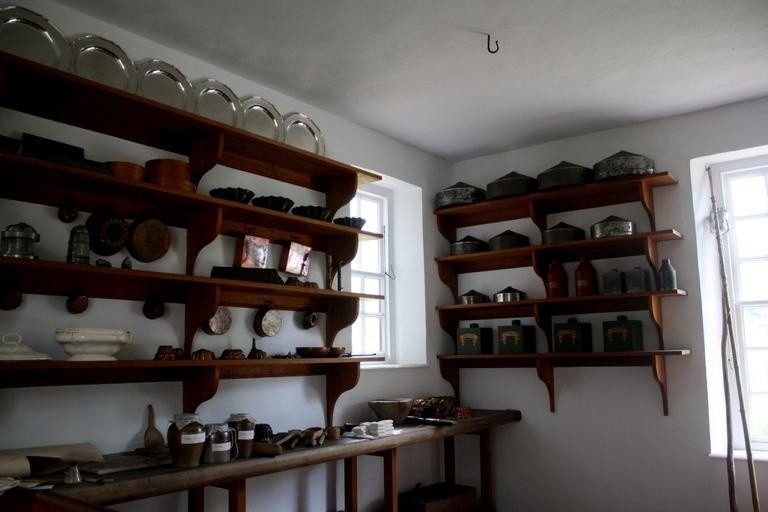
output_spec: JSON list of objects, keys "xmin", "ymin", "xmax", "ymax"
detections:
[{"xmin": 359, "ymin": 420, "xmax": 394, "ymax": 436}]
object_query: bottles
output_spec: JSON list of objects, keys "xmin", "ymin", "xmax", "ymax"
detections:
[
  {"xmin": 546, "ymin": 253, "xmax": 678, "ymax": 294},
  {"xmin": 163, "ymin": 409, "xmax": 274, "ymax": 467}
]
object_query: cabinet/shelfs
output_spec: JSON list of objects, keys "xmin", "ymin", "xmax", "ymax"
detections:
[
  {"xmin": 191, "ymin": 110, "xmax": 385, "ymax": 431},
  {"xmin": 2, "ymin": 50, "xmax": 190, "ymax": 423},
  {"xmin": 433, "ymin": 170, "xmax": 692, "ymax": 416}
]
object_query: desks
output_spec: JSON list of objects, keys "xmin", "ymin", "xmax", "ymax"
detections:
[{"xmin": 12, "ymin": 408, "xmax": 522, "ymax": 512}]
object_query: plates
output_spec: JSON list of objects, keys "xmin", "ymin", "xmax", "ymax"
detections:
[
  {"xmin": 242, "ymin": 95, "xmax": 286, "ymax": 142},
  {"xmin": 71, "ymin": 34, "xmax": 138, "ymax": 92},
  {"xmin": 137, "ymin": 59, "xmax": 196, "ymax": 113},
  {"xmin": 1, "ymin": 5, "xmax": 71, "ymax": 72},
  {"xmin": 285, "ymin": 112, "xmax": 325, "ymax": 156},
  {"xmin": 194, "ymin": 79, "xmax": 245, "ymax": 129}
]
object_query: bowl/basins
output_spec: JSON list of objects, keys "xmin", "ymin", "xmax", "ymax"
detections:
[
  {"xmin": 293, "ymin": 346, "xmax": 346, "ymax": 357},
  {"xmin": 367, "ymin": 397, "xmax": 414, "ymax": 425},
  {"xmin": 210, "ymin": 185, "xmax": 369, "ymax": 230},
  {"xmin": 154, "ymin": 343, "xmax": 248, "ymax": 359}
]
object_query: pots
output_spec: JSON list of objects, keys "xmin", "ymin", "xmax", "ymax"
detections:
[
  {"xmin": 84, "ymin": 210, "xmax": 129, "ymax": 257},
  {"xmin": 126, "ymin": 216, "xmax": 173, "ymax": 265},
  {"xmin": 255, "ymin": 299, "xmax": 284, "ymax": 340},
  {"xmin": 201, "ymin": 303, "xmax": 233, "ymax": 337}
]
